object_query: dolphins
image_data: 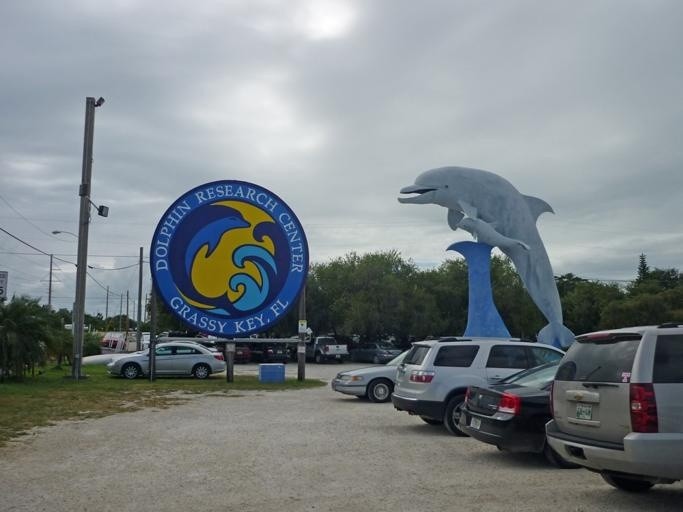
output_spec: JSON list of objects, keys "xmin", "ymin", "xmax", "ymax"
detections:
[{"xmin": 398, "ymin": 167, "xmax": 575, "ymax": 352}]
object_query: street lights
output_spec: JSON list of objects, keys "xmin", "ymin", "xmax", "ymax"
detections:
[
  {"xmin": 70, "ymin": 95, "xmax": 111, "ymax": 381},
  {"xmin": 51, "ymin": 229, "xmax": 78, "ymax": 238}
]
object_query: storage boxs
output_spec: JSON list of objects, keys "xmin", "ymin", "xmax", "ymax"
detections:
[{"xmin": 259, "ymin": 364, "xmax": 285, "ymax": 382}]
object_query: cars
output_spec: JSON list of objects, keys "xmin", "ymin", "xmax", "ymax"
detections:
[{"xmin": 101, "ymin": 330, "xmax": 298, "ymax": 379}]
